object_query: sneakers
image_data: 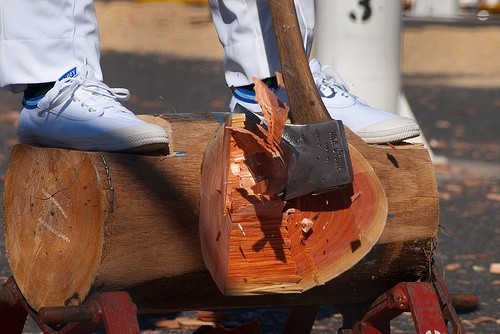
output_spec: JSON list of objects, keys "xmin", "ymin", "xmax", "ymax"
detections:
[
  {"xmin": 16, "ymin": 78, "xmax": 170, "ymax": 153},
  {"xmin": 229, "ymin": 59, "xmax": 421, "ymax": 143}
]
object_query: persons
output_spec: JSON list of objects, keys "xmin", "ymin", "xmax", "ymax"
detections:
[{"xmin": 0, "ymin": 0, "xmax": 421, "ymax": 153}]
